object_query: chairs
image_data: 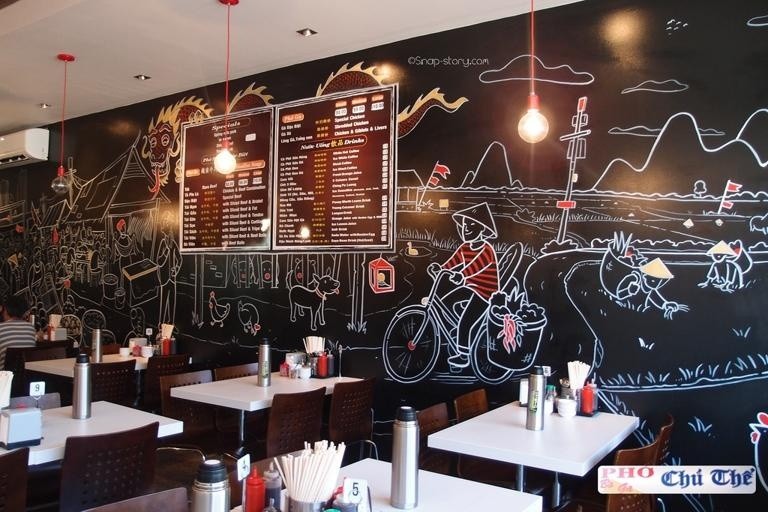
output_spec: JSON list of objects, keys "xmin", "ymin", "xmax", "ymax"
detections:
[{"xmin": 0, "ymin": 301, "xmax": 677, "ymax": 512}]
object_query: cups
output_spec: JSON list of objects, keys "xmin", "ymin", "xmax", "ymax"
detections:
[{"xmin": 285, "ymin": 492, "xmax": 325, "ymax": 512}]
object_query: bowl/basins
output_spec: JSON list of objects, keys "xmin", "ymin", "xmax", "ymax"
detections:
[{"xmin": 544, "ymin": 398, "xmax": 577, "ymax": 417}]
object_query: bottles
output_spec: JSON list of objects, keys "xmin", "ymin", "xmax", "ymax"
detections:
[
  {"xmin": 322, "ymin": 476, "xmax": 358, "ymax": 512},
  {"xmin": 190, "ymin": 459, "xmax": 232, "ymax": 512},
  {"xmin": 581, "ymin": 378, "xmax": 598, "ymax": 415},
  {"xmin": 526, "ymin": 364, "xmax": 545, "ymax": 431},
  {"xmin": 246, "ymin": 460, "xmax": 282, "ymax": 512},
  {"xmin": 390, "ymin": 405, "xmax": 420, "ymax": 509},
  {"xmin": 311, "ymin": 348, "xmax": 334, "ymax": 377},
  {"xmin": 119, "ymin": 335, "xmax": 176, "ymax": 357},
  {"xmin": 280, "ymin": 362, "xmax": 311, "ymax": 382},
  {"xmin": 257, "ymin": 337, "xmax": 271, "ymax": 387},
  {"xmin": 91, "ymin": 328, "xmax": 102, "ymax": 363},
  {"xmin": 72, "ymin": 353, "xmax": 92, "ymax": 420}
]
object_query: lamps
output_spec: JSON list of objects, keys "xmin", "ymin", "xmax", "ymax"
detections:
[
  {"xmin": 50, "ymin": 50, "xmax": 71, "ymax": 196},
  {"xmin": 214, "ymin": 0, "xmax": 237, "ymax": 175},
  {"xmin": 517, "ymin": 0, "xmax": 550, "ymax": 143}
]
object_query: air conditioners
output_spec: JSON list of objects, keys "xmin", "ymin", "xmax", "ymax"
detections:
[{"xmin": 0, "ymin": 127, "xmax": 48, "ymax": 168}]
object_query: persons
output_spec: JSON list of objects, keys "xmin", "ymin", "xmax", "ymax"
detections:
[{"xmin": 0, "ymin": 295, "xmax": 37, "ymax": 371}]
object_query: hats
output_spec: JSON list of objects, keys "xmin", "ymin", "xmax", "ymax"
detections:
[
  {"xmin": 7, "ymin": 254, "xmax": 19, "ymax": 267},
  {"xmin": 706, "ymin": 240, "xmax": 738, "ymax": 258},
  {"xmin": 452, "ymin": 202, "xmax": 498, "ymax": 239},
  {"xmin": 639, "ymin": 257, "xmax": 675, "ymax": 279}
]
